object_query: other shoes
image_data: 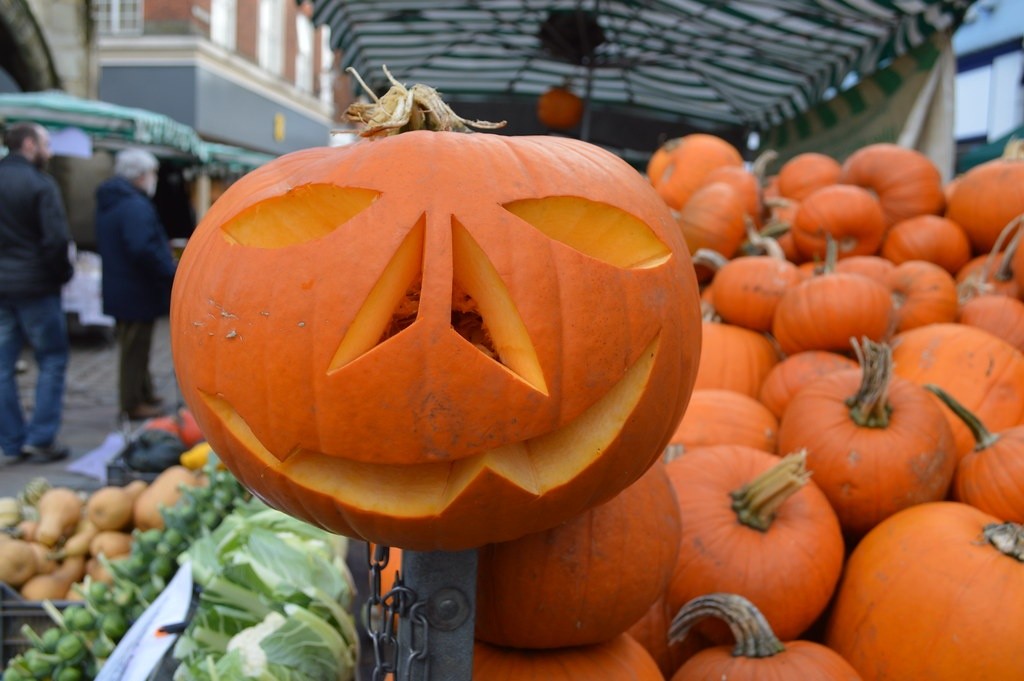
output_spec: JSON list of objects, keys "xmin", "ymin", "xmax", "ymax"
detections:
[
  {"xmin": 127, "ymin": 403, "xmax": 166, "ymax": 418},
  {"xmin": 3, "ymin": 455, "xmax": 23, "ymax": 464},
  {"xmin": 22, "ymin": 443, "xmax": 70, "ymax": 461},
  {"xmin": 148, "ymin": 391, "xmax": 165, "ymax": 402}
]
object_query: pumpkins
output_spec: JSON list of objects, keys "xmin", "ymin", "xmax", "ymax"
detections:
[
  {"xmin": 537, "ymin": 87, "xmax": 583, "ymax": 128},
  {"xmin": 373, "ymin": 132, "xmax": 1024, "ymax": 681},
  {"xmin": 0, "ymin": 409, "xmax": 224, "ymax": 602}
]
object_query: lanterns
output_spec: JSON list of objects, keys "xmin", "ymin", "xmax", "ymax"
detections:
[{"xmin": 165, "ymin": 75, "xmax": 705, "ymax": 555}]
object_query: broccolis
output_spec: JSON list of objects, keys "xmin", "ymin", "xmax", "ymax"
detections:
[{"xmin": 172, "ymin": 496, "xmax": 359, "ymax": 681}]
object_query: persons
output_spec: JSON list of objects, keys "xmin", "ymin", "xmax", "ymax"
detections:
[
  {"xmin": 0, "ymin": 120, "xmax": 75, "ymax": 466},
  {"xmin": 93, "ymin": 144, "xmax": 178, "ymax": 425}
]
